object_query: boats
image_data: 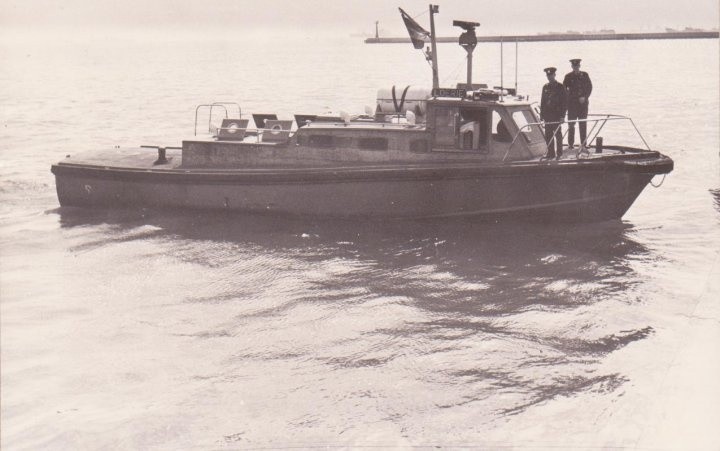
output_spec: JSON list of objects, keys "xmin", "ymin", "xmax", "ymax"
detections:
[{"xmin": 49, "ymin": 3, "xmax": 674, "ymax": 221}]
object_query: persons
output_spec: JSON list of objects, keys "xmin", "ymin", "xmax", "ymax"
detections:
[
  {"xmin": 540, "ymin": 68, "xmax": 568, "ymax": 159},
  {"xmin": 563, "ymin": 59, "xmax": 592, "ymax": 149}
]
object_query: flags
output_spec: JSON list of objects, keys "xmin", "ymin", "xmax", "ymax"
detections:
[{"xmin": 398, "ymin": 7, "xmax": 430, "ymax": 49}]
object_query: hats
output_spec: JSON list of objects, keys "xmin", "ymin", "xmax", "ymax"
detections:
[
  {"xmin": 569, "ymin": 59, "xmax": 582, "ymax": 65},
  {"xmin": 544, "ymin": 67, "xmax": 557, "ymax": 74}
]
